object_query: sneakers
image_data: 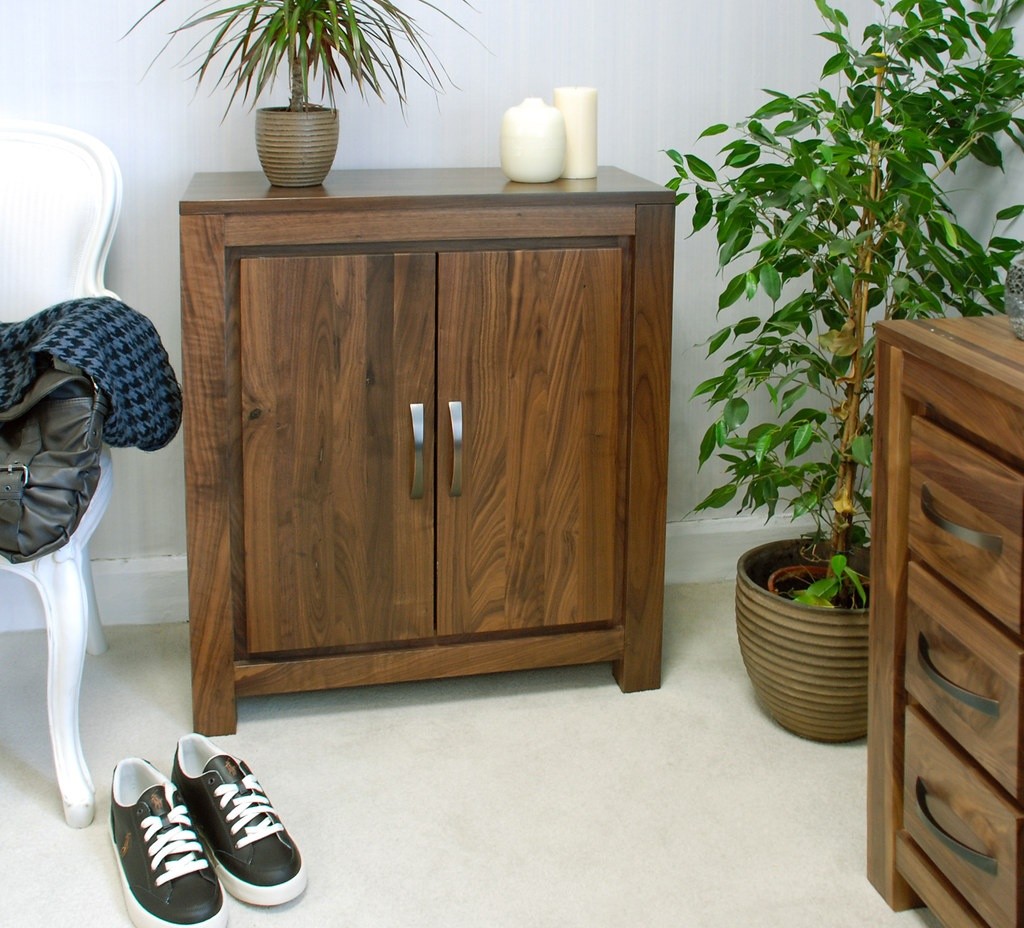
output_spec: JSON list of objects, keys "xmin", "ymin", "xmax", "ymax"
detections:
[
  {"xmin": 173, "ymin": 732, "xmax": 308, "ymax": 904},
  {"xmin": 109, "ymin": 758, "xmax": 229, "ymax": 928}
]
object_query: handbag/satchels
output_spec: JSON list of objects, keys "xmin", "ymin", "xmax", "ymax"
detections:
[{"xmin": 1, "ymin": 359, "xmax": 109, "ymax": 564}]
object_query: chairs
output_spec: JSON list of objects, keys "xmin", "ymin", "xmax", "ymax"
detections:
[{"xmin": 0, "ymin": 117, "xmax": 125, "ymax": 830}]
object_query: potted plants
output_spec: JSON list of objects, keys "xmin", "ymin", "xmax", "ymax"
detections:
[
  {"xmin": 119, "ymin": 0, "xmax": 496, "ymax": 190},
  {"xmin": 659, "ymin": 1, "xmax": 1023, "ymax": 744}
]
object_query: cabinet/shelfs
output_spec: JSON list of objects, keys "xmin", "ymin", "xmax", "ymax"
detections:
[
  {"xmin": 861, "ymin": 316, "xmax": 1023, "ymax": 928},
  {"xmin": 178, "ymin": 166, "xmax": 679, "ymax": 737}
]
